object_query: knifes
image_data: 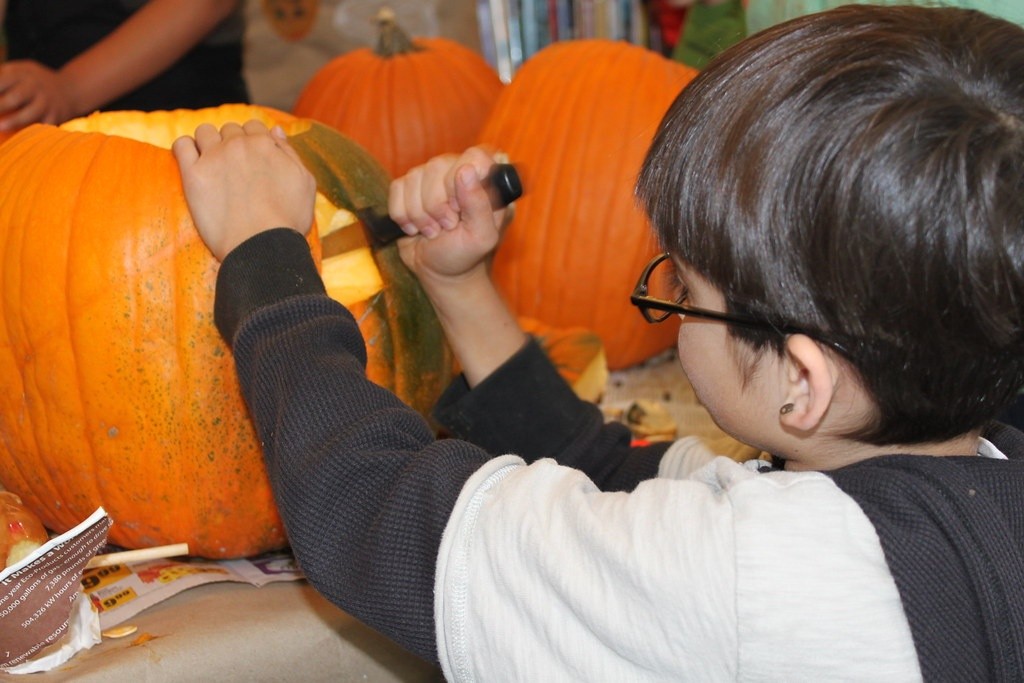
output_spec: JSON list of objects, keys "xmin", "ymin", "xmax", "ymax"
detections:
[{"xmin": 319, "ymin": 163, "xmax": 524, "ymax": 259}]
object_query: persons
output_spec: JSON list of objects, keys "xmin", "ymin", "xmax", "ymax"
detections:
[
  {"xmin": 171, "ymin": 2, "xmax": 1023, "ymax": 683},
  {"xmin": 0, "ymin": 0, "xmax": 252, "ymax": 136}
]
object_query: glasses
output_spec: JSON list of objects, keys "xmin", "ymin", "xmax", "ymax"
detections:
[{"xmin": 631, "ymin": 250, "xmax": 855, "ymax": 367}]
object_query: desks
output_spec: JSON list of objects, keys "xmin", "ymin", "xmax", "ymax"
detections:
[{"xmin": 0, "ymin": 343, "xmax": 769, "ymax": 683}]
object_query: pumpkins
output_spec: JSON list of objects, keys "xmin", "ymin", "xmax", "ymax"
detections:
[
  {"xmin": 294, "ymin": 7, "xmax": 504, "ymax": 180},
  {"xmin": 473, "ymin": 40, "xmax": 709, "ymax": 368}
]
